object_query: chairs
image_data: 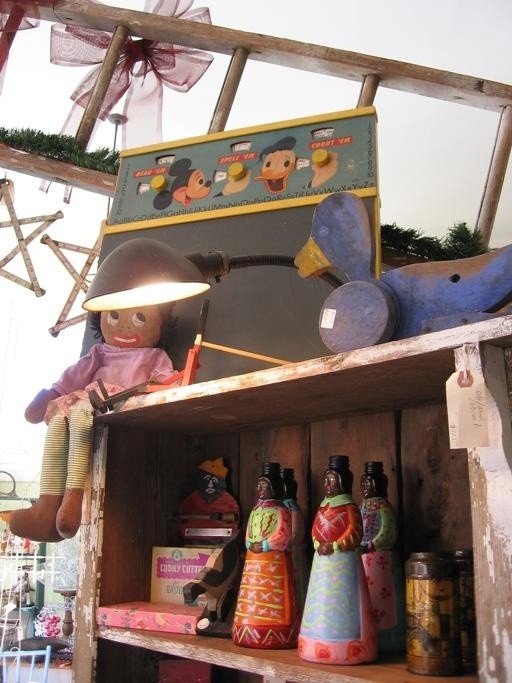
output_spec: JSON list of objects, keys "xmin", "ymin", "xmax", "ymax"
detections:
[{"xmin": 0, "ymin": 644, "xmax": 52, "ymax": 683}]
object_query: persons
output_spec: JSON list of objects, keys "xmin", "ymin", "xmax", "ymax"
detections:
[
  {"xmin": 179, "ymin": 457, "xmax": 244, "ymax": 633},
  {"xmin": 281, "ymin": 466, "xmax": 307, "ymax": 605},
  {"xmin": 297, "ymin": 454, "xmax": 381, "ymax": 663},
  {"xmin": 232, "ymin": 460, "xmax": 302, "ymax": 651},
  {"xmin": 357, "ymin": 458, "xmax": 399, "ymax": 634},
  {"xmin": 8, "ymin": 298, "xmax": 180, "ymax": 543}
]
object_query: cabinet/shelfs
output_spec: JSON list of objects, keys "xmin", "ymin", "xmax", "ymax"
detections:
[{"xmin": 73, "ymin": 318, "xmax": 510, "ymax": 682}]
